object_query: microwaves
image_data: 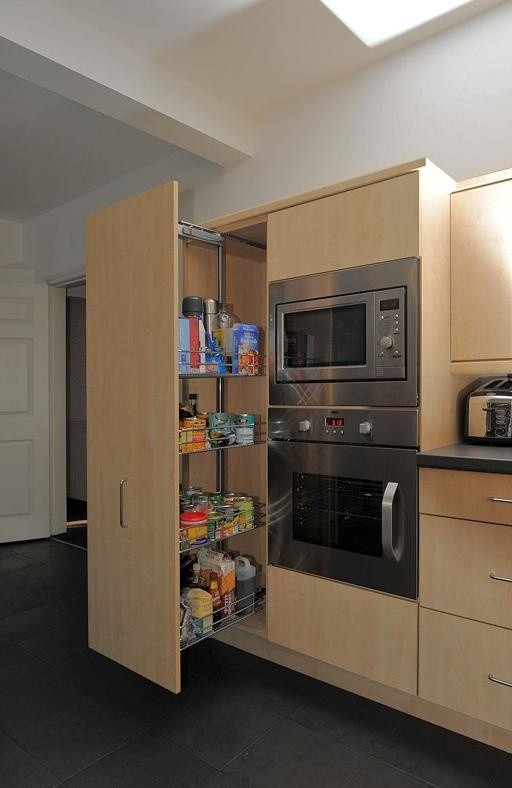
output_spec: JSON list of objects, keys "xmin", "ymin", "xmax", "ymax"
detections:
[{"xmin": 268, "ymin": 257, "xmax": 420, "ymax": 407}]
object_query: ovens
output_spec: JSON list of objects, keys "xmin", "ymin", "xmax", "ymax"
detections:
[{"xmin": 262, "ymin": 411, "xmax": 421, "ymax": 604}]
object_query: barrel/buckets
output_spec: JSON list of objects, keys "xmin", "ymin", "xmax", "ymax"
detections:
[{"xmin": 233, "ymin": 555, "xmax": 257, "ymax": 614}]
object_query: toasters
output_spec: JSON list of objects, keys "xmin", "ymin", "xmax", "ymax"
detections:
[{"xmin": 462, "ymin": 375, "xmax": 512, "ymax": 446}]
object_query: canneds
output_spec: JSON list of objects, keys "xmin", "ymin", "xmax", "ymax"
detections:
[
  {"xmin": 179, "ymin": 411, "xmax": 255, "ymax": 453},
  {"xmin": 180, "ymin": 485, "xmax": 255, "ymax": 545}
]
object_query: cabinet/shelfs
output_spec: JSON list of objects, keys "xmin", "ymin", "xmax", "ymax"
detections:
[
  {"xmin": 445, "ymin": 167, "xmax": 512, "ymax": 375},
  {"xmin": 420, "ymin": 465, "xmax": 512, "ymax": 758},
  {"xmin": 88, "ymin": 180, "xmax": 268, "ymax": 695}
]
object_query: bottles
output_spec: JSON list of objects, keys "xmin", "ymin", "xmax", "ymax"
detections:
[
  {"xmin": 187, "ymin": 393, "xmax": 198, "ymax": 419},
  {"xmin": 179, "ymin": 297, "xmax": 235, "ymax": 379},
  {"xmin": 187, "ymin": 561, "xmax": 208, "ymax": 589},
  {"xmin": 207, "ymin": 570, "xmax": 224, "ymax": 629}
]
